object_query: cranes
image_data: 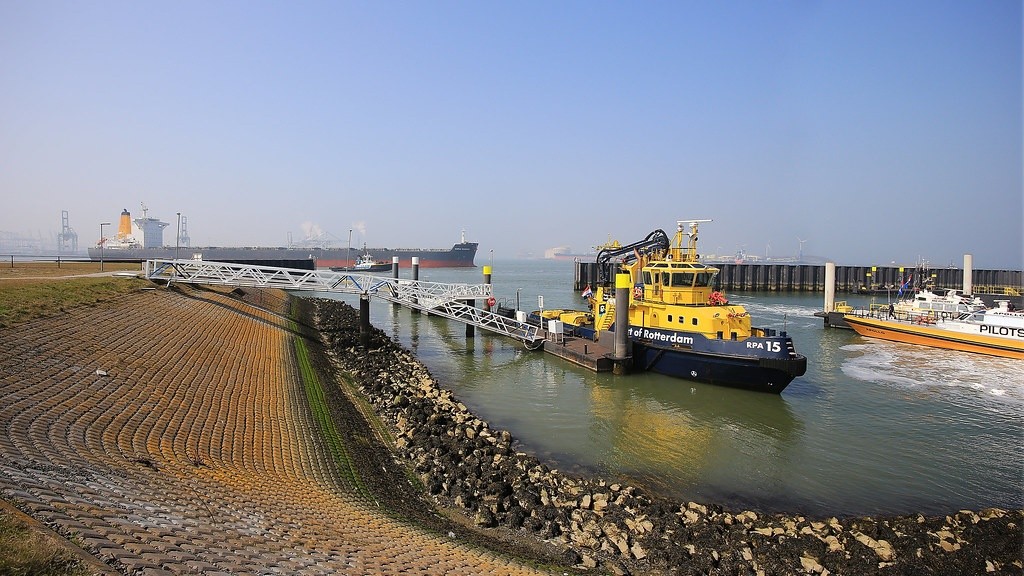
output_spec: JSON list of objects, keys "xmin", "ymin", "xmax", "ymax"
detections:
[{"xmin": 57, "ymin": 211, "xmax": 78, "ymax": 254}]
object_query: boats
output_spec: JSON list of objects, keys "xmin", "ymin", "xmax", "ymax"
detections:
[
  {"xmin": 841, "ymin": 288, "xmax": 1024, "ymax": 361},
  {"xmin": 528, "ymin": 219, "xmax": 807, "ymax": 395},
  {"xmin": 329, "ymin": 252, "xmax": 392, "ymax": 273},
  {"xmin": 834, "ymin": 301, "xmax": 853, "ymax": 313}
]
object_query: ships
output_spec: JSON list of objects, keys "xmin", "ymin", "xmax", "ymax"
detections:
[{"xmin": 87, "ymin": 231, "xmax": 479, "ymax": 268}]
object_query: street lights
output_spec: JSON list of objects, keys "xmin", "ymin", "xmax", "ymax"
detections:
[
  {"xmin": 101, "ymin": 222, "xmax": 111, "ymax": 271},
  {"xmin": 176, "ymin": 212, "xmax": 181, "ymax": 262},
  {"xmin": 489, "ymin": 248, "xmax": 494, "ymax": 270},
  {"xmin": 516, "ymin": 288, "xmax": 522, "ymax": 311}
]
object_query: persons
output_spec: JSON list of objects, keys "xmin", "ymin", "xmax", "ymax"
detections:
[
  {"xmin": 888, "ymin": 302, "xmax": 896, "ymax": 319},
  {"xmin": 1007, "ymin": 302, "xmax": 1016, "ymax": 311}
]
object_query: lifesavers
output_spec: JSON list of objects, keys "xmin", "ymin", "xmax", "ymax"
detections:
[{"xmin": 487, "ymin": 298, "xmax": 496, "ymax": 306}]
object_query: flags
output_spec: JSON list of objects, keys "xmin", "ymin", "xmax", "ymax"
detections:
[
  {"xmin": 897, "ymin": 282, "xmax": 909, "ymax": 298},
  {"xmin": 581, "ymin": 286, "xmax": 592, "ymax": 300}
]
